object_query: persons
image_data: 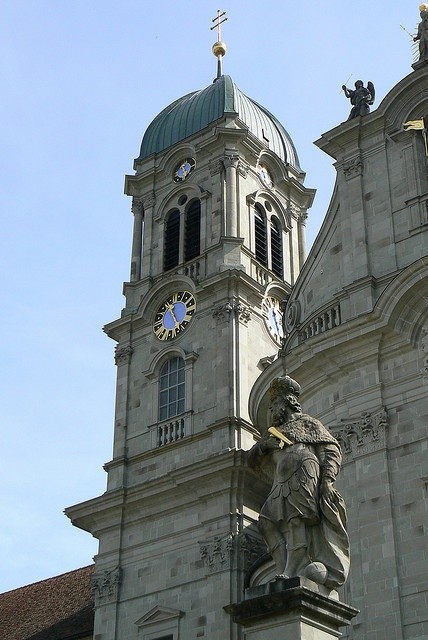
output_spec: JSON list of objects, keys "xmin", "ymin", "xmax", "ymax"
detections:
[
  {"xmin": 342, "ymin": 79, "xmax": 375, "ymax": 124},
  {"xmin": 413, "ymin": 3, "xmax": 428, "ymax": 61},
  {"xmin": 246, "ymin": 377, "xmax": 343, "ymax": 581}
]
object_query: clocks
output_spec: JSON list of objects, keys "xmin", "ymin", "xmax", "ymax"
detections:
[
  {"xmin": 172, "ymin": 157, "xmax": 195, "ymax": 184},
  {"xmin": 152, "ymin": 290, "xmax": 197, "ymax": 339},
  {"xmin": 257, "ymin": 162, "xmax": 274, "ymax": 190},
  {"xmin": 262, "ymin": 294, "xmax": 286, "ymax": 346}
]
